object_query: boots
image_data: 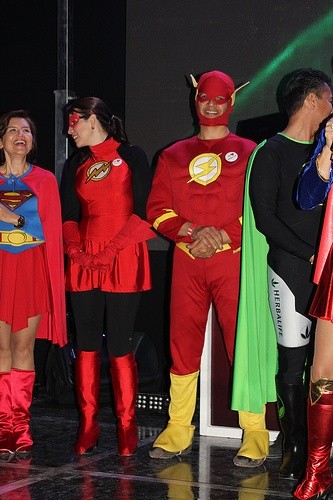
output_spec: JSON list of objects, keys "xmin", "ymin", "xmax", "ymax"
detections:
[
  {"xmin": 0, "ymin": 367, "xmax": 36, "ymax": 460},
  {"xmin": 276, "ymin": 341, "xmax": 314, "ymax": 477},
  {"xmin": 150, "ymin": 369, "xmax": 270, "ymax": 468},
  {"xmin": 294, "ymin": 365, "xmax": 333, "ymax": 500},
  {"xmin": 72, "ymin": 349, "xmax": 140, "ymax": 456}
]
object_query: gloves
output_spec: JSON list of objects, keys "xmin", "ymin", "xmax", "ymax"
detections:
[{"xmin": 61, "ymin": 213, "xmax": 142, "ymax": 273}]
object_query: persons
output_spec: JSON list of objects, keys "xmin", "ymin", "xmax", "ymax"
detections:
[
  {"xmin": 249, "ymin": 70, "xmax": 333, "ymax": 477},
  {"xmin": 146, "ymin": 71, "xmax": 268, "ymax": 467},
  {"xmin": 0, "ymin": 111, "xmax": 65, "ymax": 454},
  {"xmin": 295, "ymin": 115, "xmax": 333, "ymax": 500},
  {"xmin": 59, "ymin": 97, "xmax": 150, "ymax": 458}
]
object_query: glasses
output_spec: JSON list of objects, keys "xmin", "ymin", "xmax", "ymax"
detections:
[{"xmin": 67, "ymin": 113, "xmax": 90, "ymax": 128}]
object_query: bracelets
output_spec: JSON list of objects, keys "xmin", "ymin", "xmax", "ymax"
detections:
[{"xmin": 188, "ymin": 225, "xmax": 198, "ymax": 243}]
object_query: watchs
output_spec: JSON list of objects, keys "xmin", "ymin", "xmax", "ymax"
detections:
[{"xmin": 14, "ymin": 215, "xmax": 25, "ymax": 229}]
